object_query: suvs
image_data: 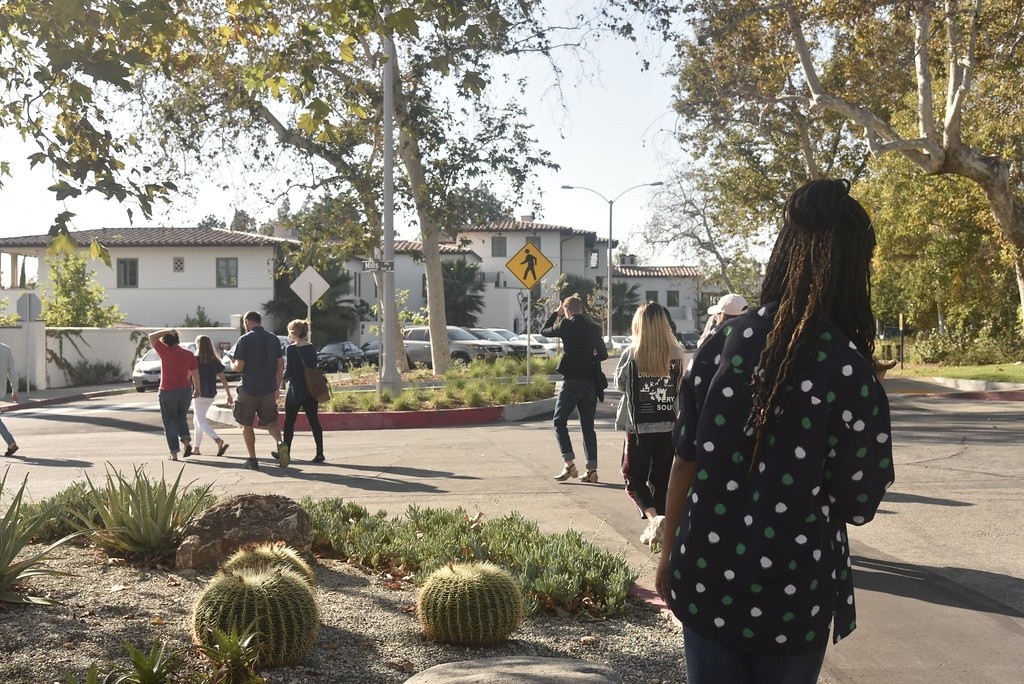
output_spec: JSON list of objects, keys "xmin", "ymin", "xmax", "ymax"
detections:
[
  {"xmin": 677, "ymin": 332, "xmax": 698, "ymax": 349},
  {"xmin": 401, "ymin": 325, "xmax": 506, "ymax": 369},
  {"xmin": 469, "ymin": 327, "xmax": 547, "ymax": 361}
]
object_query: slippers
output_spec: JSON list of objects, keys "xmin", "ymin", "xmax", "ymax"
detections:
[
  {"xmin": 192, "ymin": 452, "xmax": 200, "ymax": 455},
  {"xmin": 168, "ymin": 456, "xmax": 177, "ymax": 461},
  {"xmin": 4, "ymin": 446, "xmax": 18, "ymax": 457},
  {"xmin": 217, "ymin": 444, "xmax": 229, "ymax": 456},
  {"xmin": 183, "ymin": 445, "xmax": 192, "ymax": 457}
]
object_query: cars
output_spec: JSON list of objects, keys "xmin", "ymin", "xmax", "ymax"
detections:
[
  {"xmin": 521, "ymin": 335, "xmax": 565, "ymax": 357},
  {"xmin": 317, "ymin": 342, "xmax": 364, "ymax": 374},
  {"xmin": 221, "ymin": 335, "xmax": 292, "ymax": 382},
  {"xmin": 132, "ymin": 342, "xmax": 197, "ymax": 392},
  {"xmin": 600, "ymin": 336, "xmax": 632, "ymax": 349},
  {"xmin": 359, "ymin": 341, "xmax": 381, "ymax": 365}
]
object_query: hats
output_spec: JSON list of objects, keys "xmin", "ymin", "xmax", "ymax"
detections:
[{"xmin": 707, "ymin": 294, "xmax": 749, "ymax": 315}]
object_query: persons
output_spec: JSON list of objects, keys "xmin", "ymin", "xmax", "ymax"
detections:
[
  {"xmin": 697, "ymin": 294, "xmax": 749, "ymax": 349},
  {"xmin": 612, "ymin": 302, "xmax": 686, "ymax": 553},
  {"xmin": 230, "ymin": 311, "xmax": 290, "ymax": 469},
  {"xmin": 271, "ymin": 320, "xmax": 325, "ymax": 463},
  {"xmin": 656, "ymin": 180, "xmax": 896, "ymax": 684},
  {"xmin": 149, "ymin": 329, "xmax": 201, "ymax": 462},
  {"xmin": 188, "ymin": 335, "xmax": 233, "ymax": 456},
  {"xmin": 541, "ymin": 296, "xmax": 608, "ymax": 484},
  {"xmin": 0, "ymin": 343, "xmax": 19, "ymax": 456}
]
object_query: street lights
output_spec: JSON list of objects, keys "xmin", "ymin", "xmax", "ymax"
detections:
[{"xmin": 563, "ymin": 182, "xmax": 665, "ymax": 352}]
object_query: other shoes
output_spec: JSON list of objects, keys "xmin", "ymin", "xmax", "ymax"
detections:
[
  {"xmin": 277, "ymin": 441, "xmax": 290, "ymax": 468},
  {"xmin": 271, "ymin": 452, "xmax": 279, "ymax": 459},
  {"xmin": 640, "ymin": 524, "xmax": 652, "ymax": 544},
  {"xmin": 312, "ymin": 456, "xmax": 325, "ymax": 462},
  {"xmin": 648, "ymin": 515, "xmax": 665, "ymax": 554},
  {"xmin": 241, "ymin": 460, "xmax": 258, "ymax": 469}
]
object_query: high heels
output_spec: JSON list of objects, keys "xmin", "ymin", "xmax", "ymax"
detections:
[
  {"xmin": 553, "ymin": 464, "xmax": 578, "ymax": 481},
  {"xmin": 579, "ymin": 470, "xmax": 598, "ymax": 483}
]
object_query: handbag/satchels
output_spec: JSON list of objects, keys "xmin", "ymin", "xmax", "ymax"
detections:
[
  {"xmin": 602, "ymin": 373, "xmax": 609, "ymax": 389},
  {"xmin": 294, "ymin": 343, "xmax": 333, "ymax": 403}
]
